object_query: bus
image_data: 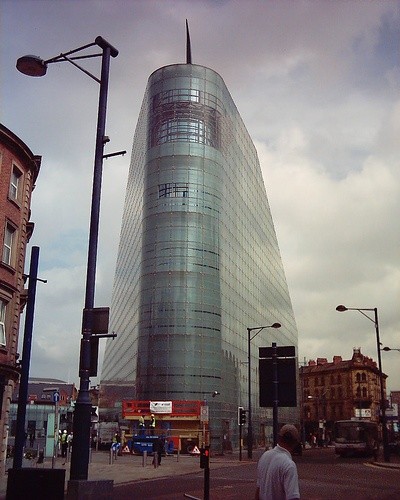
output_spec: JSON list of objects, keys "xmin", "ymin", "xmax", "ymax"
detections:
[{"xmin": 335, "ymin": 418, "xmax": 381, "ymax": 460}]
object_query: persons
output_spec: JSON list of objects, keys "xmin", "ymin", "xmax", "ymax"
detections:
[
  {"xmin": 138, "ymin": 414, "xmax": 156, "ymax": 435},
  {"xmin": 151, "ymin": 437, "xmax": 166, "ymax": 465},
  {"xmin": 255, "ymin": 425, "xmax": 301, "ymax": 500},
  {"xmin": 57, "ymin": 430, "xmax": 73, "ymax": 458},
  {"xmin": 93, "ymin": 435, "xmax": 100, "ymax": 450},
  {"xmin": 307, "ymin": 432, "xmax": 318, "ymax": 448}
]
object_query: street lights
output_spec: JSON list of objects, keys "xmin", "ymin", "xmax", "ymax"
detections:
[
  {"xmin": 335, "ymin": 305, "xmax": 390, "ymax": 461},
  {"xmin": 246, "ymin": 323, "xmax": 281, "ymax": 459},
  {"xmin": 15, "ymin": 35, "xmax": 119, "ymax": 479}
]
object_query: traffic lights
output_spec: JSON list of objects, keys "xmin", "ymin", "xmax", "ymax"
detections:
[{"xmin": 238, "ymin": 406, "xmax": 246, "ymax": 426}]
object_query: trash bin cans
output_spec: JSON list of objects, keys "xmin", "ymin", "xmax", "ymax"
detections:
[
  {"xmin": 5, "ymin": 467, "xmax": 66, "ymax": 500},
  {"xmin": 67, "ymin": 480, "xmax": 113, "ymax": 500}
]
